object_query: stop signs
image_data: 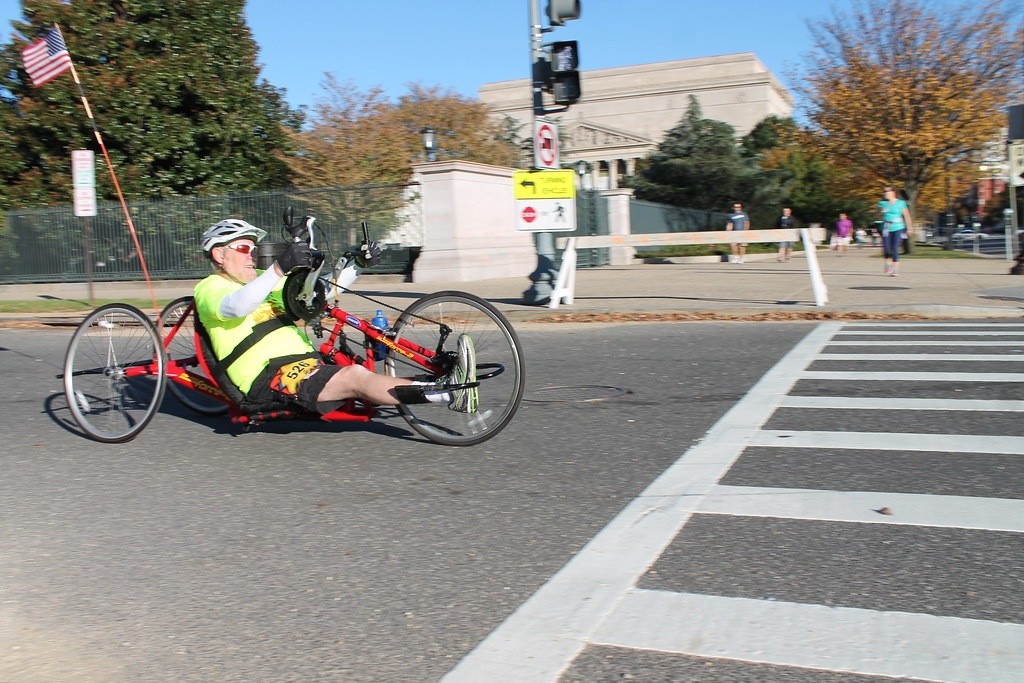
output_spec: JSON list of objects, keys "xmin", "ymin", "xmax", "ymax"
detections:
[{"xmin": 522, "ymin": 208, "xmax": 536, "ymax": 223}]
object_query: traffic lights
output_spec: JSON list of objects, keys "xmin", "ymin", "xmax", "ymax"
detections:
[
  {"xmin": 552, "ymin": 0, "xmax": 580, "ymax": 24},
  {"xmin": 552, "ymin": 40, "xmax": 580, "ymax": 104}
]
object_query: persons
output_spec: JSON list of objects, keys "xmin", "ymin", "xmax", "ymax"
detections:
[
  {"xmin": 877, "ymin": 184, "xmax": 914, "ymax": 277},
  {"xmin": 775, "ymin": 207, "xmax": 799, "ymax": 262},
  {"xmin": 725, "ymin": 203, "xmax": 751, "ymax": 264},
  {"xmin": 829, "ymin": 212, "xmax": 881, "ymax": 257},
  {"xmin": 194, "ymin": 218, "xmax": 480, "ymax": 415}
]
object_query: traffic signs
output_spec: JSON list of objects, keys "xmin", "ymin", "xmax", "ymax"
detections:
[{"xmin": 515, "ymin": 170, "xmax": 577, "ymax": 199}]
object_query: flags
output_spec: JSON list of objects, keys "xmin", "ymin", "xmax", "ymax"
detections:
[{"xmin": 21, "ymin": 27, "xmax": 72, "ymax": 87}]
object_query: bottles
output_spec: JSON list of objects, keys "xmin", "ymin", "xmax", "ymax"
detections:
[{"xmin": 372, "ymin": 308, "xmax": 388, "ymax": 361}]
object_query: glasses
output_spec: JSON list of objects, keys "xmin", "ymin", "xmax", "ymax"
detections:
[{"xmin": 221, "ymin": 243, "xmax": 258, "ymax": 257}]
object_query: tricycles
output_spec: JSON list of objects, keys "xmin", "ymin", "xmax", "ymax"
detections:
[{"xmin": 56, "ymin": 207, "xmax": 526, "ymax": 446}]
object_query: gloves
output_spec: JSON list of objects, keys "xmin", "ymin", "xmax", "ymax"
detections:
[
  {"xmin": 354, "ymin": 241, "xmax": 381, "ymax": 268},
  {"xmin": 275, "ymin": 240, "xmax": 312, "ymax": 275}
]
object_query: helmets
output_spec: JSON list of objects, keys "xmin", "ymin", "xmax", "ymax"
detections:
[{"xmin": 201, "ymin": 219, "xmax": 269, "ymax": 259}]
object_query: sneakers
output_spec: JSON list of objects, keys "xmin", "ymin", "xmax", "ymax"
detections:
[{"xmin": 445, "ymin": 334, "xmax": 479, "ymax": 413}]
